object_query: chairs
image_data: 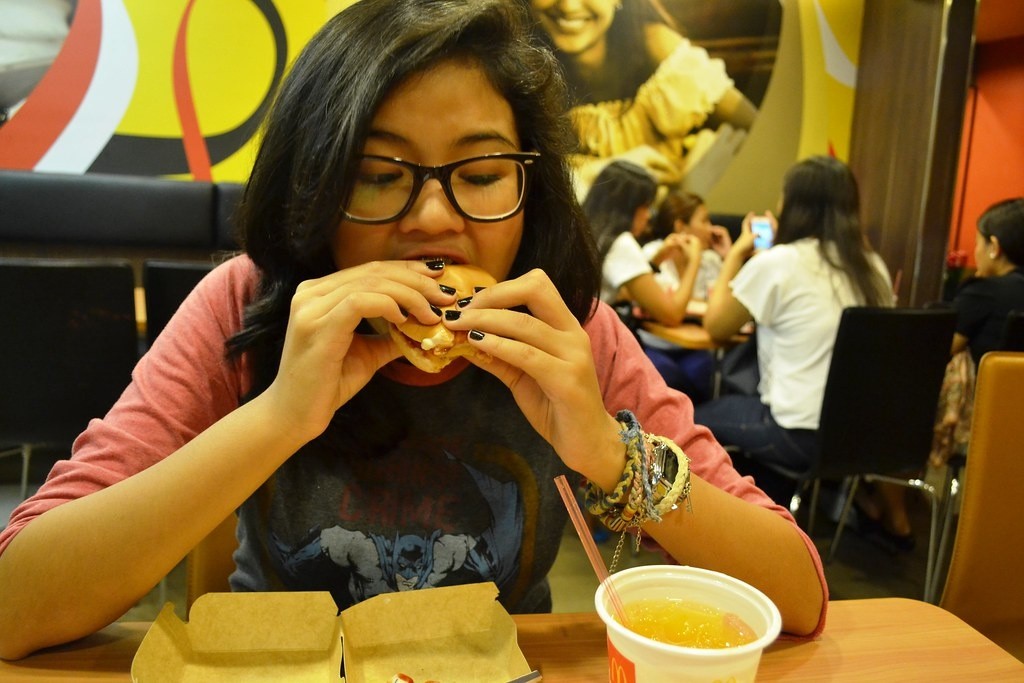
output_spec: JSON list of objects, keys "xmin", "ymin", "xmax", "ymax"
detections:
[
  {"xmin": 0, "ymin": 258, "xmax": 220, "ymax": 506},
  {"xmin": 721, "ymin": 306, "xmax": 1024, "ymax": 668}
]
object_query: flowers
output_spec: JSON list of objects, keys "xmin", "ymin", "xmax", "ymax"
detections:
[{"xmin": 943, "ymin": 250, "xmax": 969, "ymax": 303}]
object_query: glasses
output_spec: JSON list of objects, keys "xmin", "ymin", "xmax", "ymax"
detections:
[{"xmin": 337, "ymin": 148, "xmax": 541, "ymax": 224}]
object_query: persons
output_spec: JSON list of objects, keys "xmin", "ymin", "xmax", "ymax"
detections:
[
  {"xmin": 517, "ymin": 0, "xmax": 764, "ymax": 206},
  {"xmin": 0, "ymin": 30, "xmax": 830, "ymax": 658},
  {"xmin": 934, "ymin": 197, "xmax": 1024, "ymax": 365},
  {"xmin": 576, "ymin": 155, "xmax": 914, "ymax": 547}
]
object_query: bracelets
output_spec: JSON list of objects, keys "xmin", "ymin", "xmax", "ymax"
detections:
[{"xmin": 586, "ymin": 409, "xmax": 689, "ymax": 572}]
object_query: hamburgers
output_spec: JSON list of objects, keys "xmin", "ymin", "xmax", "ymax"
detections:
[{"xmin": 388, "ymin": 262, "xmax": 500, "ymax": 374}]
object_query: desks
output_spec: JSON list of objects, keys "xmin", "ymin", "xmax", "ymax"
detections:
[
  {"xmin": 638, "ymin": 313, "xmax": 757, "ymax": 398},
  {"xmin": 0, "ymin": 597, "xmax": 1024, "ymax": 683}
]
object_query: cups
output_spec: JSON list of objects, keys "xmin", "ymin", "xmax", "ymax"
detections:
[{"xmin": 595, "ymin": 565, "xmax": 781, "ymax": 683}]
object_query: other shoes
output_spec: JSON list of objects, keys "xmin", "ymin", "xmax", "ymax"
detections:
[
  {"xmin": 876, "ymin": 523, "xmax": 916, "ymax": 551},
  {"xmin": 847, "ymin": 493, "xmax": 881, "ymax": 527}
]
